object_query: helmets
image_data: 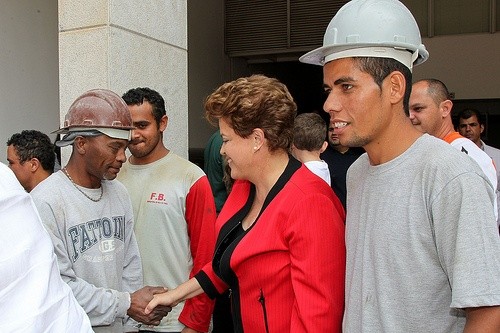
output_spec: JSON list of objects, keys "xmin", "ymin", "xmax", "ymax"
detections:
[
  {"xmin": 299, "ymin": 0, "xmax": 429, "ymax": 67},
  {"xmin": 50, "ymin": 89, "xmax": 137, "ymax": 140}
]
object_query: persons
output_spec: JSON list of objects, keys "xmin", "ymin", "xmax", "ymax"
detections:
[
  {"xmin": 144, "ymin": 75, "xmax": 346, "ymax": 333},
  {"xmin": 5, "ymin": 130, "xmax": 55, "ymax": 193},
  {"xmin": 28, "ymin": 88, "xmax": 172, "ymax": 333},
  {"xmin": 116, "ymin": 87, "xmax": 217, "ymax": 333},
  {"xmin": 300, "ymin": 0, "xmax": 500, "ymax": 333},
  {"xmin": 204, "ymin": 79, "xmax": 500, "ymax": 231}
]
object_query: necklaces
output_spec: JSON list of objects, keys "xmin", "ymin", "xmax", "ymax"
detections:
[{"xmin": 63, "ymin": 167, "xmax": 104, "ymax": 203}]
